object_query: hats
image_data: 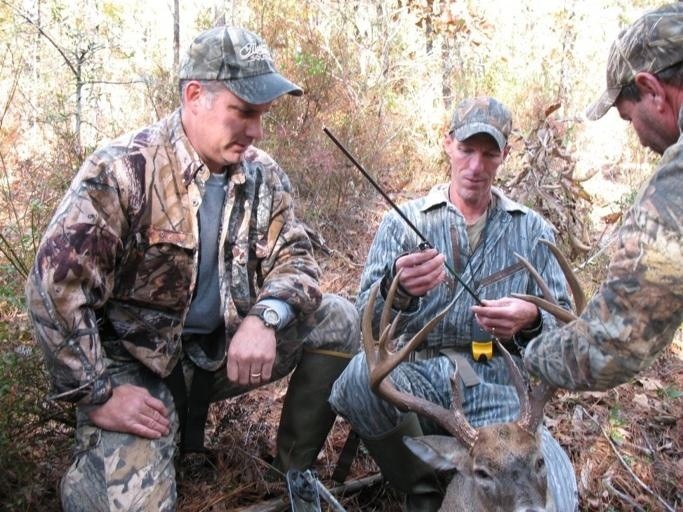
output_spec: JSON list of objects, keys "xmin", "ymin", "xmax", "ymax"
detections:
[
  {"xmin": 584, "ymin": 2, "xmax": 682, "ymax": 122},
  {"xmin": 446, "ymin": 95, "xmax": 513, "ymax": 153},
  {"xmin": 178, "ymin": 25, "xmax": 303, "ymax": 106}
]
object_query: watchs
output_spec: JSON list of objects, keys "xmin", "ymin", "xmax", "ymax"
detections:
[{"xmin": 247, "ymin": 308, "xmax": 280, "ymax": 333}]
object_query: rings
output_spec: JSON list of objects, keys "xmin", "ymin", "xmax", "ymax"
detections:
[{"xmin": 250, "ymin": 373, "xmax": 261, "ymax": 377}]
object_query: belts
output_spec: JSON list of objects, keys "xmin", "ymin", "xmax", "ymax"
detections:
[{"xmin": 408, "ymin": 338, "xmax": 522, "ymax": 363}]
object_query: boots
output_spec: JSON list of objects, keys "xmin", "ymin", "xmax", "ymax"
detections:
[
  {"xmin": 350, "ymin": 411, "xmax": 444, "ymax": 512},
  {"xmin": 274, "ymin": 345, "xmax": 357, "ymax": 480}
]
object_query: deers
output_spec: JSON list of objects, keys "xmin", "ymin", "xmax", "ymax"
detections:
[{"xmin": 361, "ymin": 237, "xmax": 589, "ymax": 512}]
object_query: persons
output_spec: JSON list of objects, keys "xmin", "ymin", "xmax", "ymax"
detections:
[
  {"xmin": 328, "ymin": 97, "xmax": 579, "ymax": 512},
  {"xmin": 523, "ymin": 0, "xmax": 683, "ymax": 392},
  {"xmin": 24, "ymin": 26, "xmax": 359, "ymax": 512}
]
